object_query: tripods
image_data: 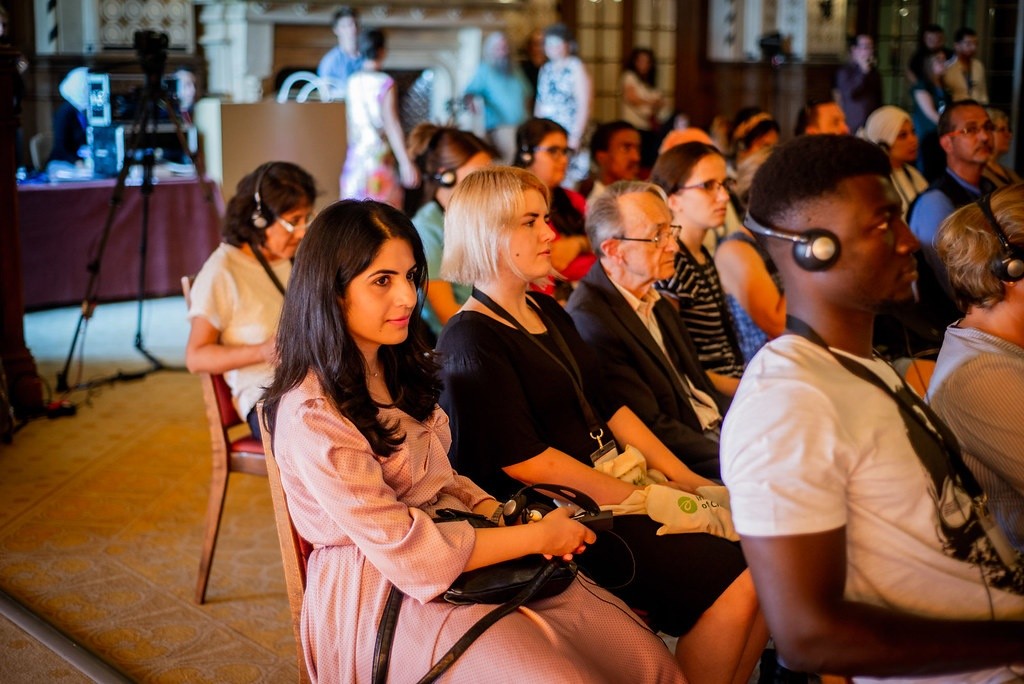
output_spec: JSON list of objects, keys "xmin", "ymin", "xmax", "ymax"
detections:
[{"xmin": 56, "ymin": 86, "xmax": 218, "ymax": 394}]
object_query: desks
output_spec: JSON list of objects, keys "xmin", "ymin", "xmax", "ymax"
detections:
[{"xmin": 19, "ymin": 177, "xmax": 226, "ymax": 312}]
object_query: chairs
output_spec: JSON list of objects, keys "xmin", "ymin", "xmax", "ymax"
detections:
[
  {"xmin": 255, "ymin": 400, "xmax": 309, "ymax": 684},
  {"xmin": 180, "ymin": 277, "xmax": 268, "ymax": 605}
]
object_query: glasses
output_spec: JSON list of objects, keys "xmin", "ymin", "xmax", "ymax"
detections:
[
  {"xmin": 682, "ymin": 176, "xmax": 737, "ymax": 198},
  {"xmin": 530, "ymin": 144, "xmax": 576, "ymax": 160},
  {"xmin": 945, "ymin": 122, "xmax": 995, "ymax": 137},
  {"xmin": 616, "ymin": 225, "xmax": 683, "ymax": 250},
  {"xmin": 274, "ymin": 214, "xmax": 315, "ymax": 232}
]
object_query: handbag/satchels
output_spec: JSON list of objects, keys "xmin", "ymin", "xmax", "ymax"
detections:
[{"xmin": 428, "ymin": 507, "xmax": 573, "ymax": 604}]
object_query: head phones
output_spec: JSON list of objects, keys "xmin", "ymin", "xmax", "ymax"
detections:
[
  {"xmin": 976, "ymin": 195, "xmax": 1023, "ymax": 281},
  {"xmin": 249, "ymin": 161, "xmax": 275, "ymax": 230},
  {"xmin": 423, "ymin": 169, "xmax": 456, "ymax": 188},
  {"xmin": 744, "ymin": 208, "xmax": 841, "ymax": 272},
  {"xmin": 519, "ymin": 124, "xmax": 534, "ymax": 165},
  {"xmin": 503, "ymin": 482, "xmax": 600, "ymax": 524}
]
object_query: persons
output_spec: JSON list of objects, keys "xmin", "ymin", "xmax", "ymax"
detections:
[{"xmin": 184, "ymin": 8, "xmax": 1024, "ymax": 683}]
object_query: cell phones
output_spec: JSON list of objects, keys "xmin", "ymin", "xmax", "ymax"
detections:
[{"xmin": 572, "ymin": 509, "xmax": 614, "ymax": 533}]
